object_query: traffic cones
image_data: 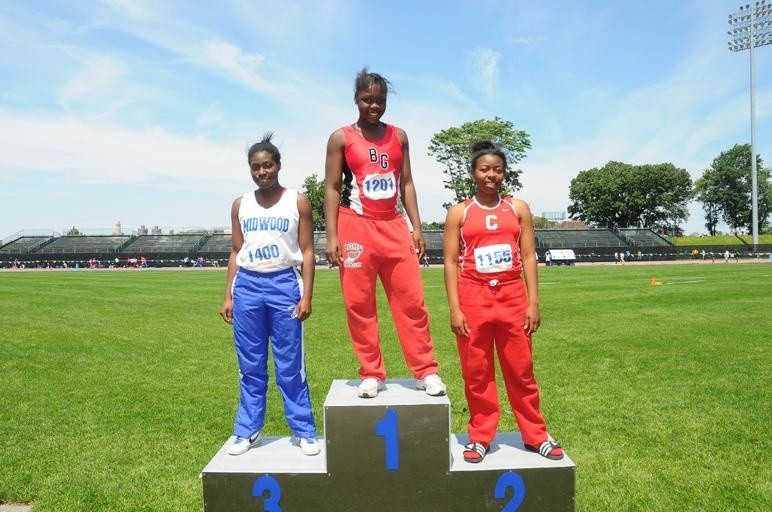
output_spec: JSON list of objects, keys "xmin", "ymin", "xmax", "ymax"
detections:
[{"xmin": 649, "ymin": 277, "xmax": 657, "ymax": 286}]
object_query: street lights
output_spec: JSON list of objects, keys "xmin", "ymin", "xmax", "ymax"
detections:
[{"xmin": 725, "ymin": 2, "xmax": 771, "ymax": 255}]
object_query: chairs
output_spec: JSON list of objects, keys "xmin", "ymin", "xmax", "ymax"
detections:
[
  {"xmin": 1, "ymin": 233, "xmax": 232, "ymax": 258},
  {"xmin": 536, "ymin": 226, "xmax": 676, "ymax": 256},
  {"xmin": 312, "ymin": 230, "xmax": 331, "ymax": 251},
  {"xmin": 412, "ymin": 230, "xmax": 447, "ymax": 253}
]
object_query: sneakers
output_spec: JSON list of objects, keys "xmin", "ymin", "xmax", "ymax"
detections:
[
  {"xmin": 358, "ymin": 377, "xmax": 386, "ymax": 397},
  {"xmin": 290, "ymin": 431, "xmax": 321, "ymax": 456},
  {"xmin": 414, "ymin": 373, "xmax": 447, "ymax": 396},
  {"xmin": 226, "ymin": 430, "xmax": 264, "ymax": 454},
  {"xmin": 524, "ymin": 438, "xmax": 564, "ymax": 459},
  {"xmin": 462, "ymin": 439, "xmax": 489, "ymax": 461}
]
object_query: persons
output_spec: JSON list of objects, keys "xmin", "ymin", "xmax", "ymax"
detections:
[
  {"xmin": 637, "ymin": 249, "xmax": 641, "ymax": 261},
  {"xmin": 614, "ymin": 249, "xmax": 635, "ymax": 264},
  {"xmin": 735, "ymin": 250, "xmax": 739, "ymax": 263},
  {"xmin": 220, "ymin": 127, "xmax": 323, "ymax": 456},
  {"xmin": 545, "ymin": 253, "xmax": 551, "ymax": 266},
  {"xmin": 710, "ymin": 249, "xmax": 716, "ymax": 263},
  {"xmin": 183, "ymin": 255, "xmax": 204, "ymax": 267},
  {"xmin": 443, "ymin": 140, "xmax": 565, "ymax": 463},
  {"xmin": 421, "ymin": 253, "xmax": 429, "ymax": 267},
  {"xmin": 724, "ymin": 248, "xmax": 730, "ymax": 263},
  {"xmin": 323, "ymin": 65, "xmax": 448, "ymax": 397},
  {"xmin": 11, "ymin": 255, "xmax": 148, "ymax": 270}
]
